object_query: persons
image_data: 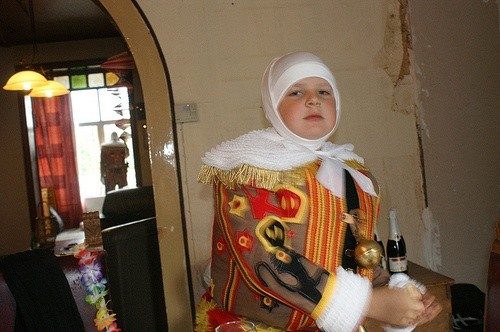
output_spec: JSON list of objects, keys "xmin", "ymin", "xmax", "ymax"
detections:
[
  {"xmin": 101, "ymin": 134, "xmax": 130, "ymax": 197},
  {"xmin": 197, "ymin": 50, "xmax": 444, "ymax": 332}
]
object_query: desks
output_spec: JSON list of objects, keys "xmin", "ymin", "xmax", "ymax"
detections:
[{"xmin": 349, "ymin": 257, "xmax": 455, "ymax": 332}]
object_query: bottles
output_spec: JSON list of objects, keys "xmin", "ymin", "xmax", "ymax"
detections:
[
  {"xmin": 387, "ymin": 208, "xmax": 408, "ymax": 274},
  {"xmin": 374, "ymin": 225, "xmax": 385, "ymax": 259}
]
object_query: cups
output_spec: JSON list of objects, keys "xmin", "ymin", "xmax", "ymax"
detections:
[{"xmin": 215, "ymin": 321, "xmax": 257, "ymax": 332}]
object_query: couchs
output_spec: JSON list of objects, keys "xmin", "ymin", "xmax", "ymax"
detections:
[{"xmin": 0, "ymin": 215, "xmax": 169, "ymax": 332}]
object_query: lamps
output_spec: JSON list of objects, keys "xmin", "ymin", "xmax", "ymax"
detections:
[{"xmin": 3, "ymin": 0, "xmax": 71, "ymax": 97}]
